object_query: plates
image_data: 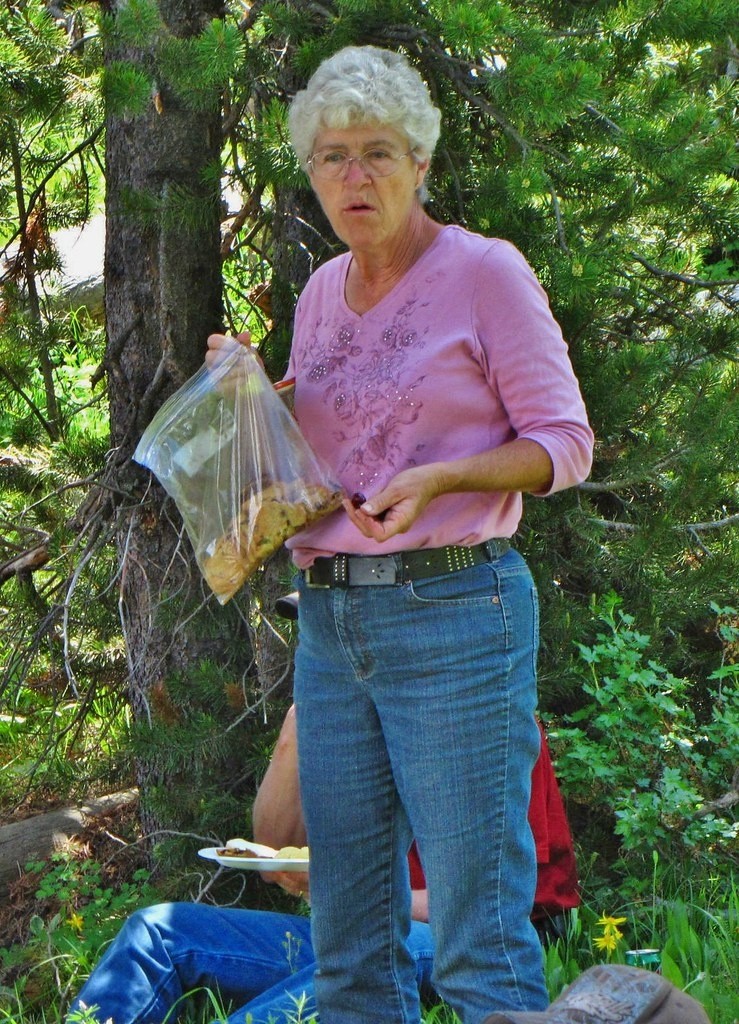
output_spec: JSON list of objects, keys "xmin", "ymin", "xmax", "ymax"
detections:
[{"xmin": 199, "ymin": 846, "xmax": 309, "ymax": 870}]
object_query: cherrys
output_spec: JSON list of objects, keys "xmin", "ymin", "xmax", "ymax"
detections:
[{"xmin": 351, "ymin": 492, "xmax": 366, "ymax": 509}]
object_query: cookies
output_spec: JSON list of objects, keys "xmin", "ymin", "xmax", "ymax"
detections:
[
  {"xmin": 216, "ymin": 848, "xmax": 256, "ymax": 858},
  {"xmin": 202, "ymin": 472, "xmax": 350, "ymax": 596}
]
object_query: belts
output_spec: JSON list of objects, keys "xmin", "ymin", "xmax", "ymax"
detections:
[{"xmin": 299, "ymin": 537, "xmax": 511, "ymax": 588}]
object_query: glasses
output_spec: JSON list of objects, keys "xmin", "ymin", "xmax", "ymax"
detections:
[{"xmin": 305, "ymin": 148, "xmax": 418, "ymax": 180}]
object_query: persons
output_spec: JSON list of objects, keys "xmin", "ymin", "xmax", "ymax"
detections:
[
  {"xmin": 204, "ymin": 46, "xmax": 596, "ymax": 1024},
  {"xmin": 70, "ymin": 702, "xmax": 581, "ymax": 1024}
]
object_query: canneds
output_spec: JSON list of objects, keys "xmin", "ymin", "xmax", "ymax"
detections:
[{"xmin": 625, "ymin": 948, "xmax": 666, "ymax": 978}]
